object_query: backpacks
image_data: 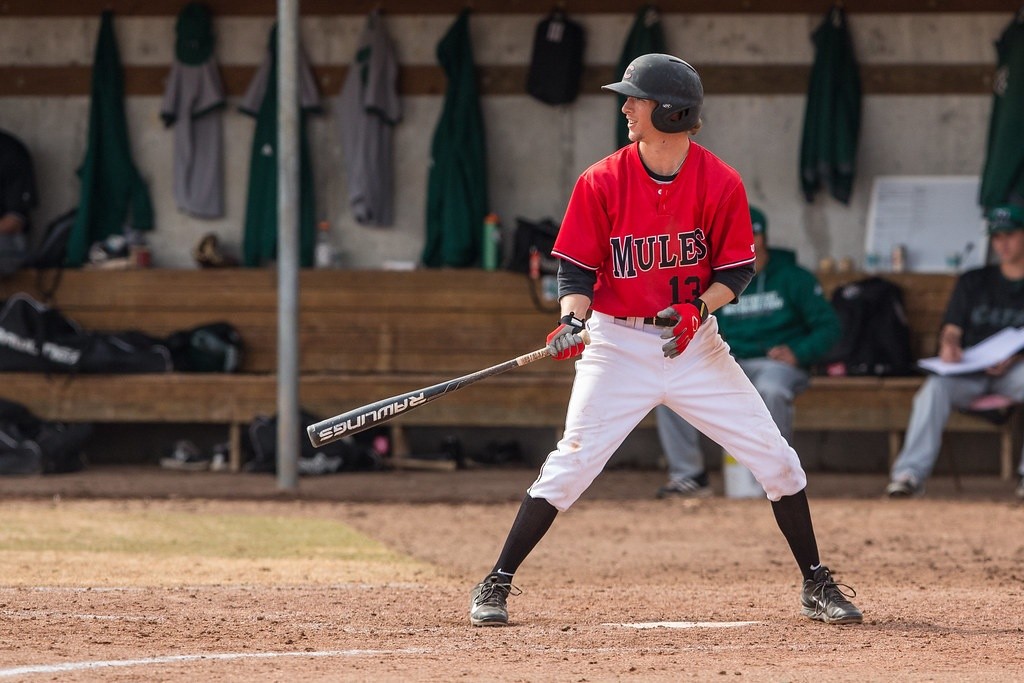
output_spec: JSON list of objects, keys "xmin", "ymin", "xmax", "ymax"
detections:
[{"xmin": 819, "ymin": 277, "xmax": 924, "ymax": 375}]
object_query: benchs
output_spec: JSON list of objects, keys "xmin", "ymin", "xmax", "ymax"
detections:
[{"xmin": 1, "ymin": 268, "xmax": 1023, "ymax": 479}]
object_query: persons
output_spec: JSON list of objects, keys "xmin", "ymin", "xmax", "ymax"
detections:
[
  {"xmin": 654, "ymin": 206, "xmax": 848, "ymax": 498},
  {"xmin": 0, "ymin": 129, "xmax": 34, "ymax": 284},
  {"xmin": 469, "ymin": 54, "xmax": 862, "ymax": 625},
  {"xmin": 884, "ymin": 203, "xmax": 1024, "ymax": 500}
]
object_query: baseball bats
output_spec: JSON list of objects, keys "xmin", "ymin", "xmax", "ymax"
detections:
[{"xmin": 306, "ymin": 328, "xmax": 592, "ymax": 449}]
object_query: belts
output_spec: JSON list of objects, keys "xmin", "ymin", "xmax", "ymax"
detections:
[{"xmin": 615, "ymin": 316, "xmax": 678, "ymax": 327}]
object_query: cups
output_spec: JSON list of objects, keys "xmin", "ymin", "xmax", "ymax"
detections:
[{"xmin": 134, "ymin": 249, "xmax": 151, "ymax": 268}]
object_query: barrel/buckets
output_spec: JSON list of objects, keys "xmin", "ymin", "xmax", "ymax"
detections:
[{"xmin": 721, "ymin": 452, "xmax": 769, "ymax": 498}]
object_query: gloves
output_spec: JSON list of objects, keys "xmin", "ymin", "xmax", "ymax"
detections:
[
  {"xmin": 657, "ymin": 303, "xmax": 702, "ymax": 359},
  {"xmin": 545, "ymin": 311, "xmax": 585, "ymax": 360}
]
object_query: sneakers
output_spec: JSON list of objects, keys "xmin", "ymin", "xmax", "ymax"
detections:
[
  {"xmin": 885, "ymin": 471, "xmax": 928, "ymax": 499},
  {"xmin": 800, "ymin": 567, "xmax": 863, "ymax": 624},
  {"xmin": 657, "ymin": 473, "xmax": 713, "ymax": 499},
  {"xmin": 469, "ymin": 571, "xmax": 523, "ymax": 626}
]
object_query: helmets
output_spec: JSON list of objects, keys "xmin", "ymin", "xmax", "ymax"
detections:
[{"xmin": 600, "ymin": 53, "xmax": 704, "ymax": 133}]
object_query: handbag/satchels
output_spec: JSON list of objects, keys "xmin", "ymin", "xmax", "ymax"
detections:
[{"xmin": 503, "ymin": 216, "xmax": 562, "ymax": 315}]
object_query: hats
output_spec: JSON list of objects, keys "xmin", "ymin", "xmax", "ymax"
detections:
[
  {"xmin": 986, "ymin": 203, "xmax": 1024, "ymax": 233},
  {"xmin": 748, "ymin": 206, "xmax": 768, "ymax": 235}
]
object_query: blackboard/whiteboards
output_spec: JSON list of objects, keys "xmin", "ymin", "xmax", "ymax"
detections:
[{"xmin": 863, "ymin": 175, "xmax": 988, "ymax": 274}]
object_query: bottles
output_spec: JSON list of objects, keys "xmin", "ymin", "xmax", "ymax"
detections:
[
  {"xmin": 313, "ymin": 222, "xmax": 333, "ymax": 269},
  {"xmin": 480, "ymin": 211, "xmax": 503, "ymax": 272}
]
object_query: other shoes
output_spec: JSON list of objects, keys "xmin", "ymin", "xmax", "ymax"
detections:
[{"xmin": 157, "ymin": 438, "xmax": 231, "ymax": 472}]
